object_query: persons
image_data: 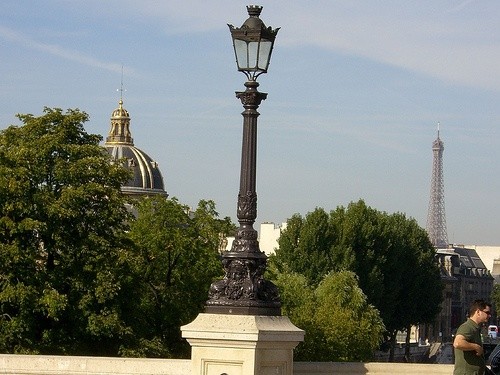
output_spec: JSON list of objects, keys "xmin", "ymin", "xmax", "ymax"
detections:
[{"xmin": 452, "ymin": 298, "xmax": 492, "ymax": 375}]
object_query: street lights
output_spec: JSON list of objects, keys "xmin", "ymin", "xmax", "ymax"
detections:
[{"xmin": 202, "ymin": 4, "xmax": 284, "ymax": 315}]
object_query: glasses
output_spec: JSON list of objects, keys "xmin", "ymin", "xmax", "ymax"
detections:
[{"xmin": 480, "ymin": 309, "xmax": 492, "ymax": 316}]
